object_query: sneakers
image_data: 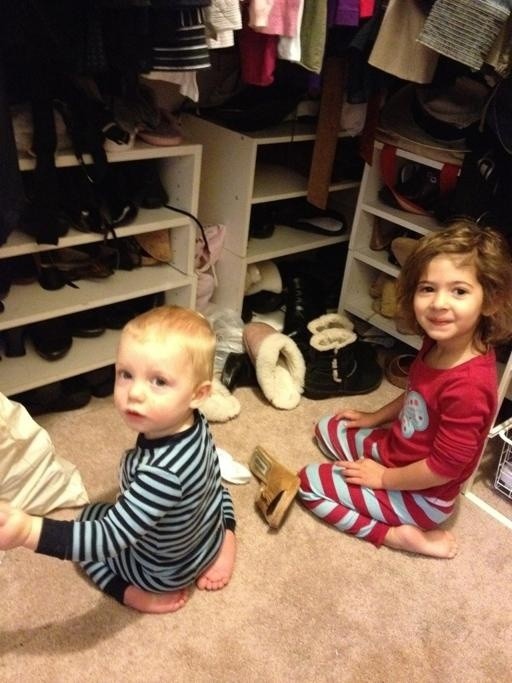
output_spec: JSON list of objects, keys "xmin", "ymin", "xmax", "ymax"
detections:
[
  {"xmin": 243, "ymin": 195, "xmax": 361, "ymax": 343},
  {"xmin": 365, "ymin": 147, "xmax": 493, "ymax": 396}
]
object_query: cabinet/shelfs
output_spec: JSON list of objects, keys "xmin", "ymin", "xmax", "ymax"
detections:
[
  {"xmin": 0, "ymin": 140, "xmax": 205, "ymax": 399},
  {"xmin": 332, "ymin": 136, "xmax": 462, "ymax": 354},
  {"xmin": 182, "ymin": 113, "xmax": 358, "ymax": 334}
]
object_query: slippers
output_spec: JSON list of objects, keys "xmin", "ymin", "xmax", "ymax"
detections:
[
  {"xmin": 242, "ymin": 318, "xmax": 308, "ymax": 411},
  {"xmin": 248, "ymin": 448, "xmax": 302, "ymax": 530}
]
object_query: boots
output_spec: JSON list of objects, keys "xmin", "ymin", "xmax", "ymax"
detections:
[{"xmin": 303, "ymin": 313, "xmax": 385, "ymax": 400}]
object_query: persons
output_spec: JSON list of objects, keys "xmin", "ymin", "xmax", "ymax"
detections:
[
  {"xmin": 283, "ymin": 214, "xmax": 512, "ymax": 560},
  {"xmin": 0, "ymin": 304, "xmax": 239, "ymax": 619}
]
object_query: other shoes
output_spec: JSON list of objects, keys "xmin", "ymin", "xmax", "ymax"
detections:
[{"xmin": 0, "ymin": 84, "xmax": 182, "ymax": 419}]
